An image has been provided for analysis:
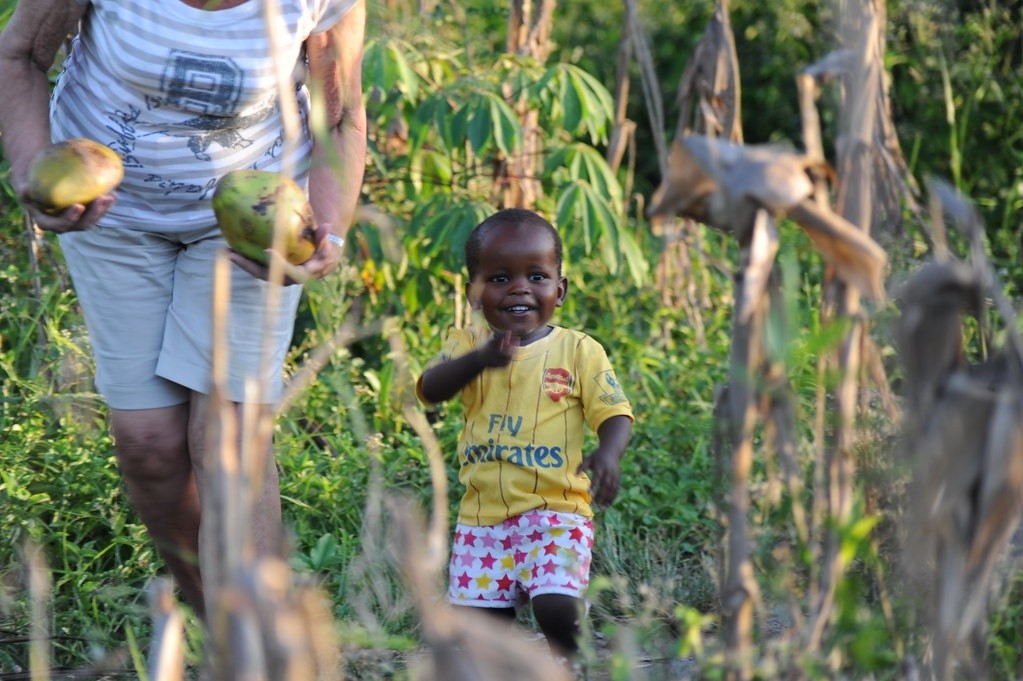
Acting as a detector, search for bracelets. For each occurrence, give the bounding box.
[329,233,346,248]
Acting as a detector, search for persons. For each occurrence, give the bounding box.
[414,207,635,681]
[1,0,369,681]
